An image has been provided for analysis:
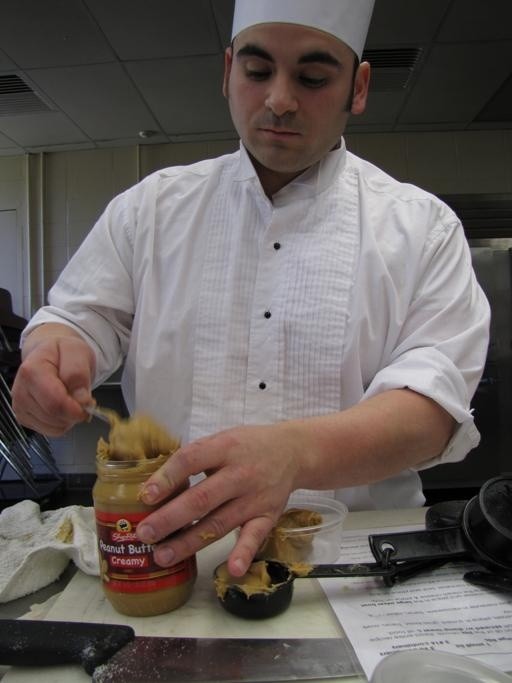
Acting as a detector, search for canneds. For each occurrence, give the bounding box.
[89,447,200,618]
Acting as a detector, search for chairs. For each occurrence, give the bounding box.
[0,288,67,503]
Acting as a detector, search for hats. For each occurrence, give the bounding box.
[231,0,377,65]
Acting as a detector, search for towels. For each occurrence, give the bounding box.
[0,498,101,605]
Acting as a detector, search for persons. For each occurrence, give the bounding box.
[11,22,493,576]
